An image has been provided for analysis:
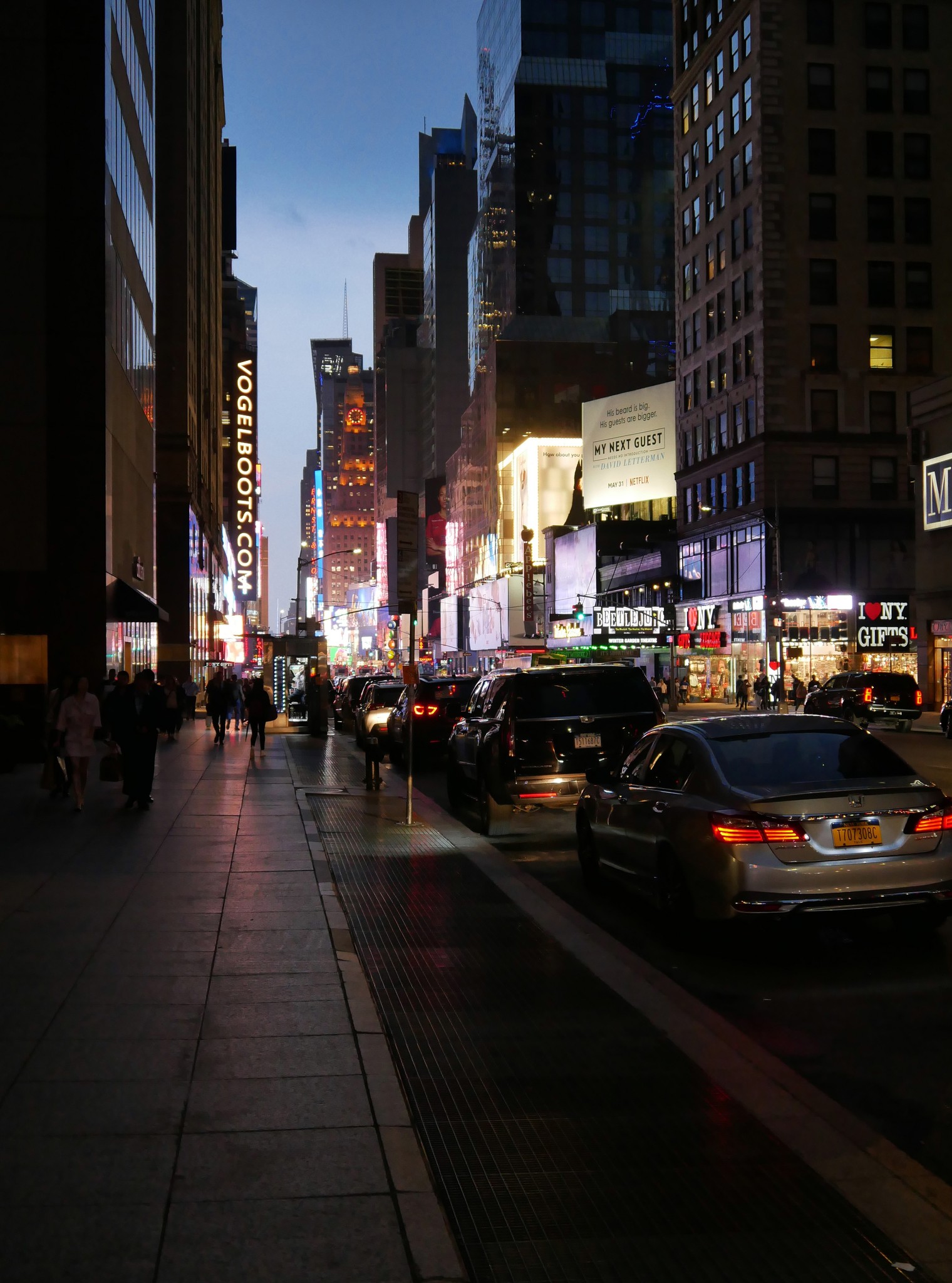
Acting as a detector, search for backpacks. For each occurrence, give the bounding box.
[228,686,238,701]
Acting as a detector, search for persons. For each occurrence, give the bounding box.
[96,669,186,811]
[736,675,743,707]
[663,676,690,706]
[649,677,657,694]
[182,675,199,721]
[56,676,102,811]
[754,675,772,710]
[772,678,781,709]
[657,679,667,703]
[808,675,821,693]
[245,678,271,758]
[288,666,309,719]
[739,680,752,710]
[204,671,236,746]
[203,672,257,730]
[365,724,385,789]
[791,674,800,706]
[795,681,808,713]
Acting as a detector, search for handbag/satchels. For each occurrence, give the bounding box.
[206,703,211,716]
[42,757,68,791]
[100,752,124,782]
[265,704,277,722]
[205,715,212,728]
[167,691,177,708]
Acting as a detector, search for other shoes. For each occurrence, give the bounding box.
[219,740,224,745]
[260,750,266,757]
[214,732,220,743]
[50,791,58,799]
[250,748,254,758]
[226,720,230,730]
[241,724,246,727]
[62,789,70,797]
[234,726,239,730]
[74,797,84,810]
[138,796,154,810]
[125,795,135,809]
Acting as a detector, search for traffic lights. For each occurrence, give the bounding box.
[786,646,803,658]
[572,604,583,621]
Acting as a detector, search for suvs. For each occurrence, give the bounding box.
[386,672,484,788]
[332,672,406,752]
[803,669,925,733]
[448,661,668,837]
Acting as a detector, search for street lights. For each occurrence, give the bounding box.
[295,542,362,636]
[466,596,504,668]
[504,574,547,650]
[697,503,790,714]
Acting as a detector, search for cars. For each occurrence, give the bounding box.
[939,700,952,739]
[576,713,952,964]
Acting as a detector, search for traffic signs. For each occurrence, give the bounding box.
[497,646,506,650]
[463,653,472,656]
[665,630,681,636]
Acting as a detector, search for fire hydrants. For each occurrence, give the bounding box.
[362,736,390,792]
